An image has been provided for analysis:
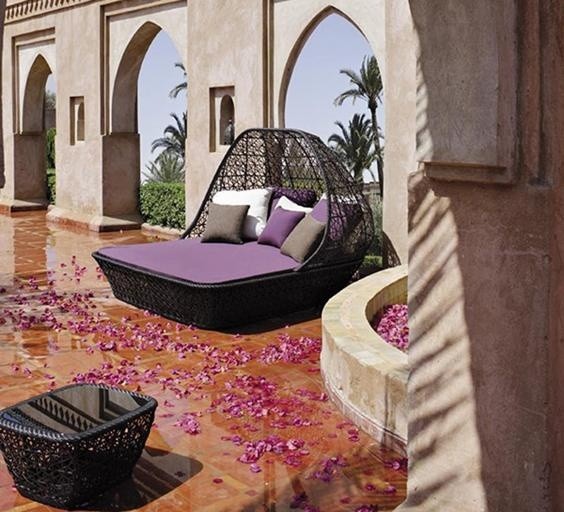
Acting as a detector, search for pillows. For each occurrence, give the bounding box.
[199,182,368,263]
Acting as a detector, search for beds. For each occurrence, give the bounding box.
[90,129,374,330]
